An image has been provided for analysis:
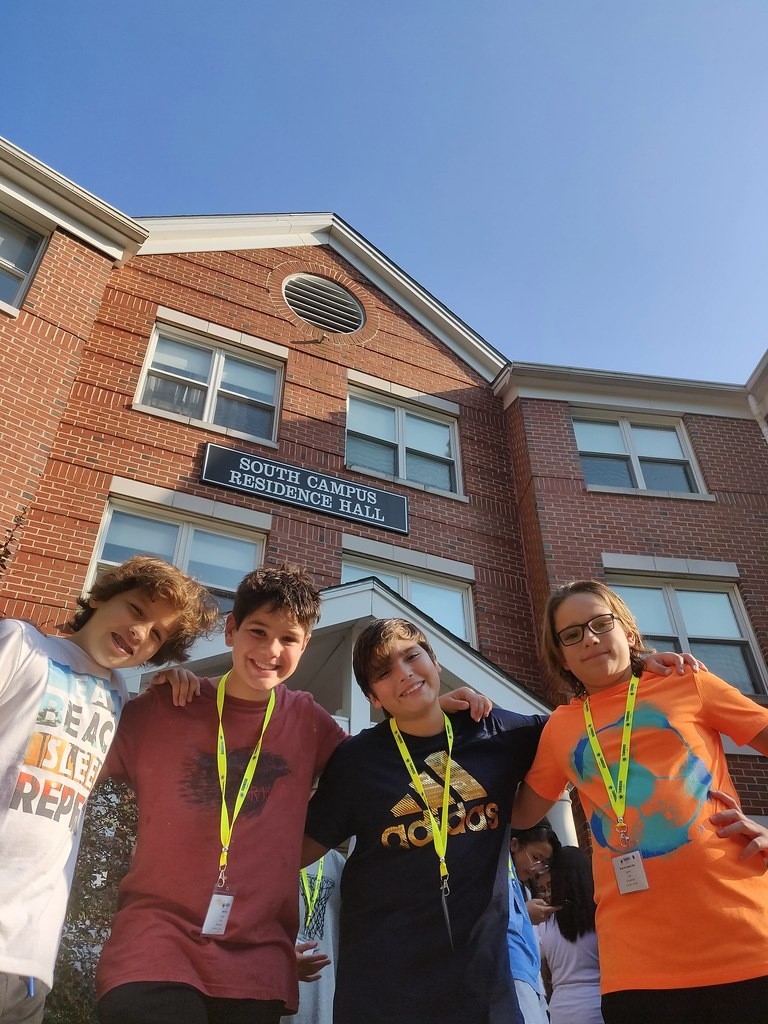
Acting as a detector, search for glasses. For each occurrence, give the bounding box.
[521,845,551,876]
[556,612,627,646]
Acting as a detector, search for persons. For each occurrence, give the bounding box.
[509,825,603,1024]
[280,770,346,1023]
[0,556,219,1024]
[95,565,492,1024]
[510,581,768,1024]
[300,618,709,1024]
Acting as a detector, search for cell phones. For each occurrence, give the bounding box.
[547,899,572,906]
